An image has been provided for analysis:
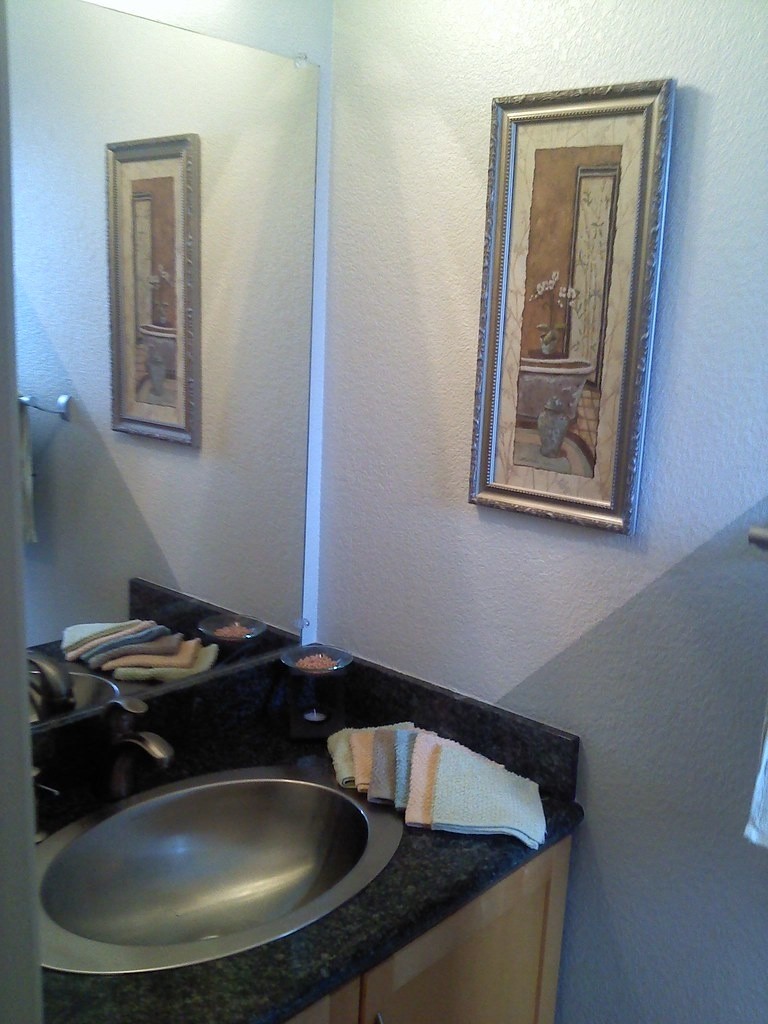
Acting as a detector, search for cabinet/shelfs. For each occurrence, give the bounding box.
[284,832,574,1024]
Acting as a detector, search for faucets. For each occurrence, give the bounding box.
[97,695,174,797]
[29,651,77,718]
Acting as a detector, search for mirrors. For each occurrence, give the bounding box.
[1,0,320,729]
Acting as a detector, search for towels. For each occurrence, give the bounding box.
[60,617,219,681]
[327,728,354,787]
[431,742,547,848]
[367,727,393,803]
[393,724,414,810]
[21,393,36,544]
[403,729,507,831]
[352,729,374,792]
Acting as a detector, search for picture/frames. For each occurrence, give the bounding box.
[105,133,203,448]
[466,77,677,536]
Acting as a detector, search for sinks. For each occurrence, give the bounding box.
[36,766,404,975]
[31,669,119,720]
